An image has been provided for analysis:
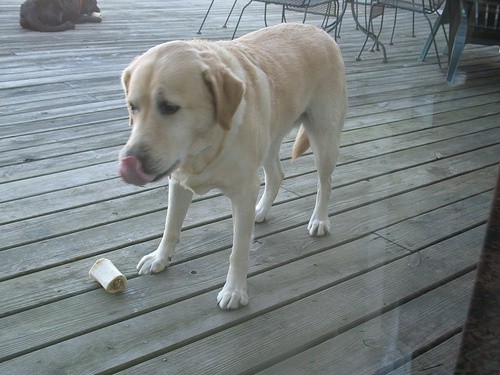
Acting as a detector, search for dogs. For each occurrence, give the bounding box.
[117,22,344,311]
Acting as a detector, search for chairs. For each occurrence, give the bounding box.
[195,0,500,86]
